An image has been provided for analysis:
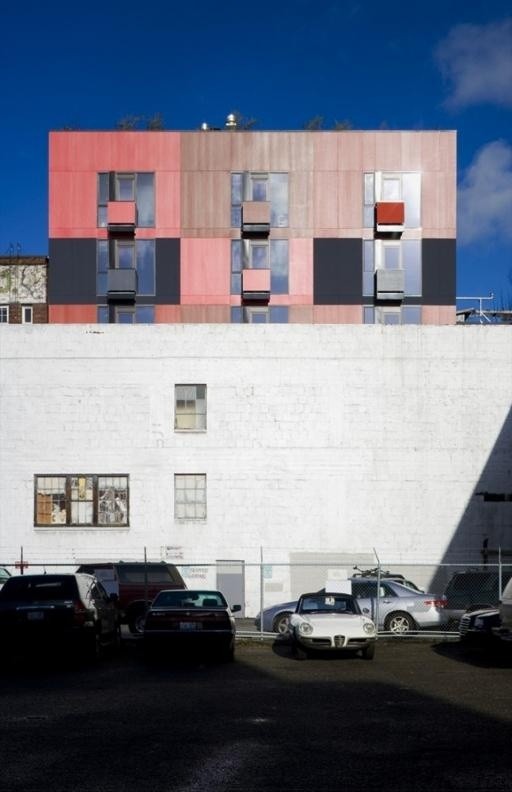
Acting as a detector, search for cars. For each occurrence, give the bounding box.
[256,565,512,661]
[0,561,235,665]
